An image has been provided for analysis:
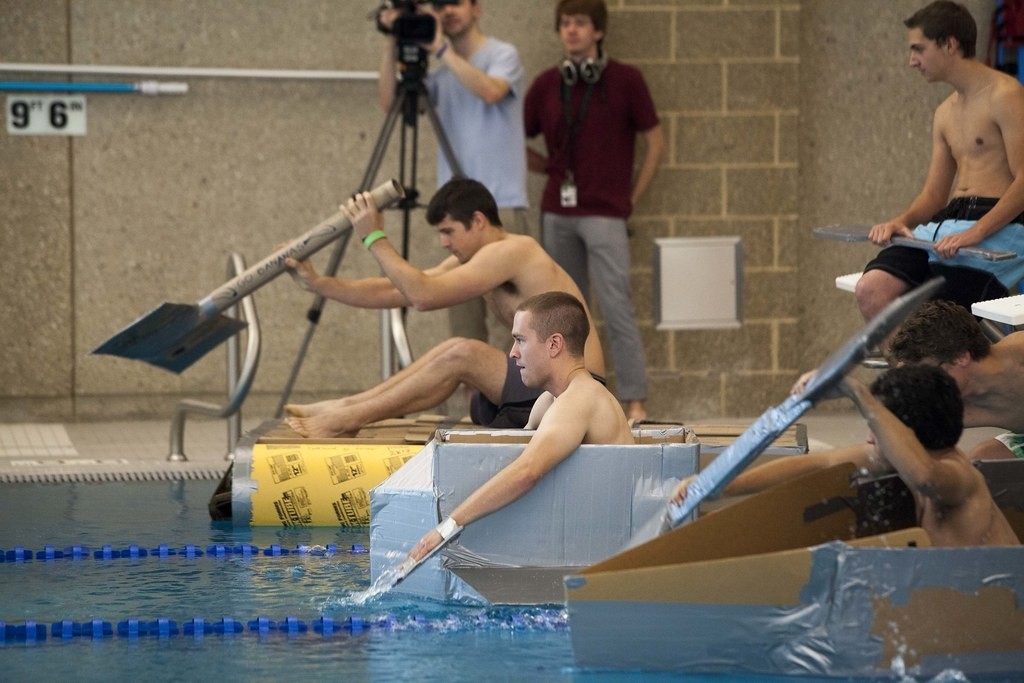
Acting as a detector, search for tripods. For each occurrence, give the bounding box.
[273,45,465,420]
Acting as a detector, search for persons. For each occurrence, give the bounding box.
[395,291,635,569]
[523,0,664,427]
[890,301,1024,459]
[275,178,607,439]
[855,0,1024,369]
[670,364,1021,547]
[378,0,530,403]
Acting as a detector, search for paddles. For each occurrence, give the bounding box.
[813,223,1017,263]
[619,275,946,554]
[88,175,408,379]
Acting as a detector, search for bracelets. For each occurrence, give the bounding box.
[437,44,447,59]
[365,231,386,248]
